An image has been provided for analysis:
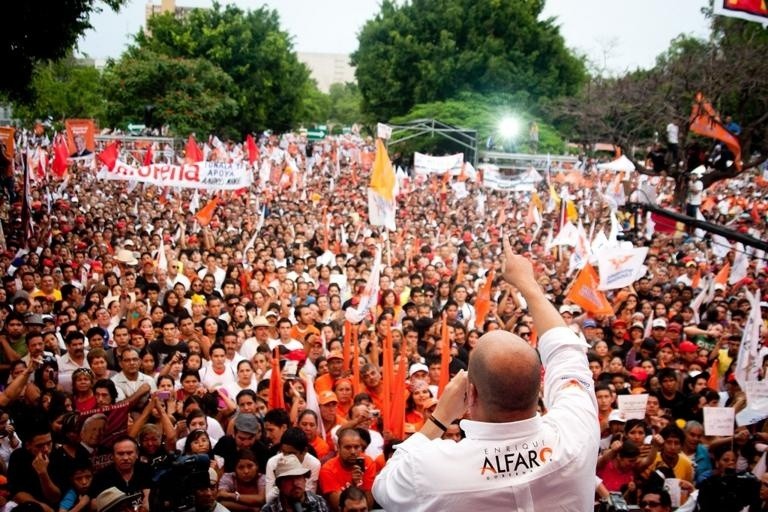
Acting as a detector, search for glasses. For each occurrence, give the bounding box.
[639,500,663,508]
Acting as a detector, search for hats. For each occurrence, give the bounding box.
[191,294,208,307]
[246,311,280,331]
[559,304,597,329]
[628,320,644,331]
[316,390,339,406]
[409,362,429,377]
[652,318,667,329]
[273,455,311,484]
[326,350,345,361]
[608,409,628,422]
[234,413,261,434]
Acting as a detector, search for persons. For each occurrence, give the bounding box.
[0,113,768,511]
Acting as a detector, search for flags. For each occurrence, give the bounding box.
[687,91,744,173]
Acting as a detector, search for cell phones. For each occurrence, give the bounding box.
[356,457,364,470]
[48,368,54,380]
[159,391,171,400]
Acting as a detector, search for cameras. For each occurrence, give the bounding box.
[180,353,188,361]
[371,410,380,418]
